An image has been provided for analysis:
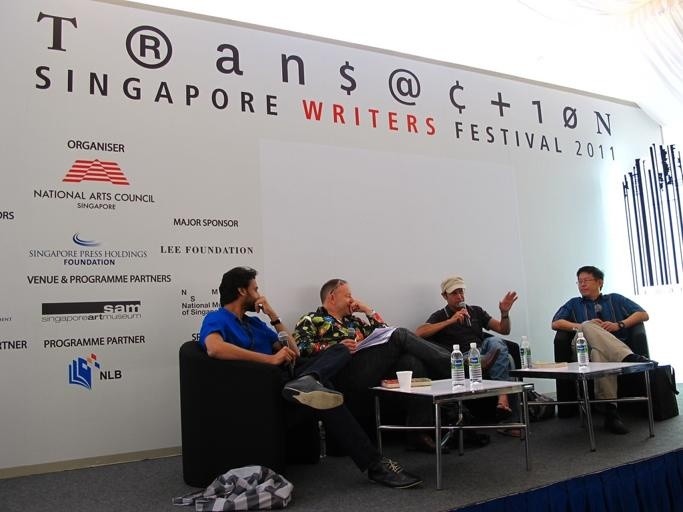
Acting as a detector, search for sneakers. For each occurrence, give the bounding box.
[496,421,529,436]
[497,402,513,415]
[636,355,658,370]
[603,413,630,434]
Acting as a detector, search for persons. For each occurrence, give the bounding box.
[291,278,501,451]
[551,265,659,434]
[416,275,532,437]
[199,265,425,490]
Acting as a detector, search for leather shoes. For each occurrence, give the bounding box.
[366,456,423,489]
[417,433,450,456]
[283,376,344,411]
[465,348,500,376]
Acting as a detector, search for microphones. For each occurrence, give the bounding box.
[459,302,472,329]
[348,328,356,339]
[594,304,605,322]
[278,331,294,380]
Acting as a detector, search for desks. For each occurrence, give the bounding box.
[369,377,534,490]
[509,361,656,452]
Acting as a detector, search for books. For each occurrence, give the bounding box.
[380,377,432,387]
[530,360,568,369]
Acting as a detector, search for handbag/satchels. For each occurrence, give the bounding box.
[522,385,555,421]
[172,464,293,511]
[635,365,679,421]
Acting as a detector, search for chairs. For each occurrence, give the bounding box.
[554,318,651,420]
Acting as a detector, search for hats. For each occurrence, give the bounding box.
[441,276,467,295]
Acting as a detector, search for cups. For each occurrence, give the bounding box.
[396,371,413,391]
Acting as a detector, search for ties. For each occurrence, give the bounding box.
[593,300,603,320]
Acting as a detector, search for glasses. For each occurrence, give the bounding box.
[576,279,594,285]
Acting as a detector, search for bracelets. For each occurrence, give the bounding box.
[501,316,508,318]
[270,317,281,325]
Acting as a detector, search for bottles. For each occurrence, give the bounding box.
[576,332,589,367]
[468,343,483,384]
[450,344,466,388]
[518,335,532,369]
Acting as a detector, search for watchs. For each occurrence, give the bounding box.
[617,321,625,329]
